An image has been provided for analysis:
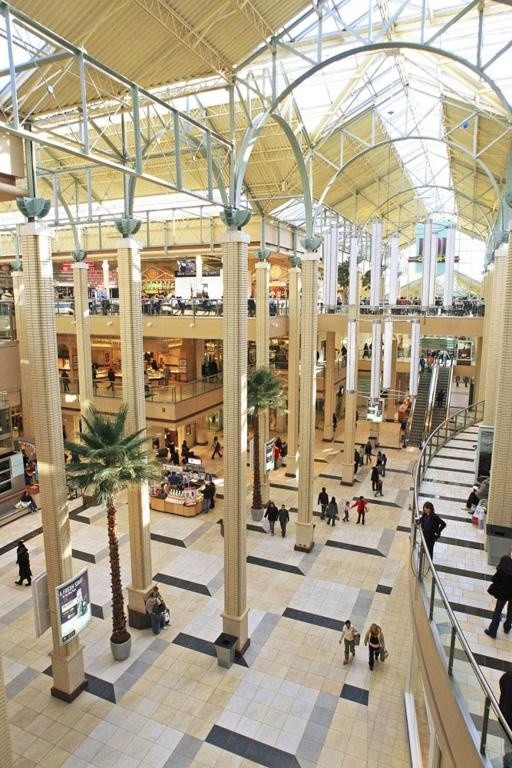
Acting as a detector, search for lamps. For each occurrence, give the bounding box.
[320,79,458,407]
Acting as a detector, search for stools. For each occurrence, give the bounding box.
[26,484,39,494]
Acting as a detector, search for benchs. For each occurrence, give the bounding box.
[0,488,33,527]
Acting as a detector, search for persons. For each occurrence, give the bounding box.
[154,586,171,630]
[339,620,359,665]
[364,624,385,671]
[415,501,446,567]
[14,540,33,586]
[484,552,512,639]
[145,592,161,635]
[498,670,512,768]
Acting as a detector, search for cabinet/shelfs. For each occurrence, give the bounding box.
[0,388,23,451]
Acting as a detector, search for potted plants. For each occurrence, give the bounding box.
[361,269,378,304]
[246,365,289,521]
[337,252,363,314]
[63,399,167,661]
